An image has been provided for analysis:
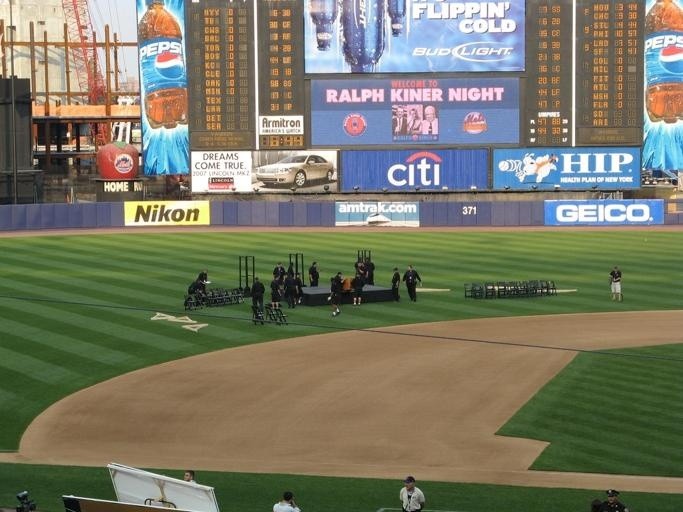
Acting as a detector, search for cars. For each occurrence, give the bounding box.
[256,154,335,187]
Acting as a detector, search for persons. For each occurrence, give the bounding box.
[419,105,438,136]
[350,257,375,305]
[403,264,421,302]
[188,268,208,306]
[399,476,425,512]
[608,266,622,302]
[392,107,407,134]
[327,277,339,317]
[591,500,603,512]
[184,470,196,483]
[391,268,401,302]
[250,277,265,319]
[407,109,420,134]
[271,262,307,314]
[272,492,301,512]
[309,261,319,287]
[603,489,627,511]
[336,272,346,291]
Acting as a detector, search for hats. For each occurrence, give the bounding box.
[606,489,618,497]
[284,492,293,499]
[403,479,413,483]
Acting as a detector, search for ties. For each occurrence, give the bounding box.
[428,122,432,133]
[411,119,415,128]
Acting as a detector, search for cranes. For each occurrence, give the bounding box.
[61,0,127,146]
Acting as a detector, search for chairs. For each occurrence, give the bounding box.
[265,305,289,326]
[251,306,264,325]
[464,279,558,299]
[184,288,246,310]
